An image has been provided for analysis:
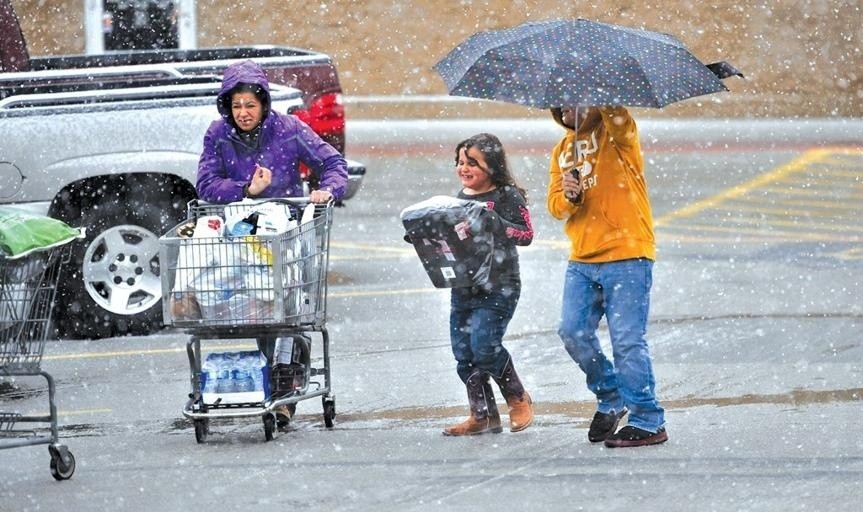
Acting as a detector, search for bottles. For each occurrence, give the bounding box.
[202,350,267,395]
[170,291,201,319]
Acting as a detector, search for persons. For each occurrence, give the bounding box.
[548,104,668,445]
[441,134,534,435]
[195,62,347,422]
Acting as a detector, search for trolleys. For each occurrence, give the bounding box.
[0,240,78,480]
[157,196,337,442]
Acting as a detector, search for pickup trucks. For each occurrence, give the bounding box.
[0,0,346,162]
[0,83,366,335]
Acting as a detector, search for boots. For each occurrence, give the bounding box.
[442,377,504,437]
[486,354,535,432]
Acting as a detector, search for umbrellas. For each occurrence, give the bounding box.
[431,14,744,201]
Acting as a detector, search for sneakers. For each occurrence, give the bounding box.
[272,403,298,429]
[603,425,670,448]
[588,408,629,444]
